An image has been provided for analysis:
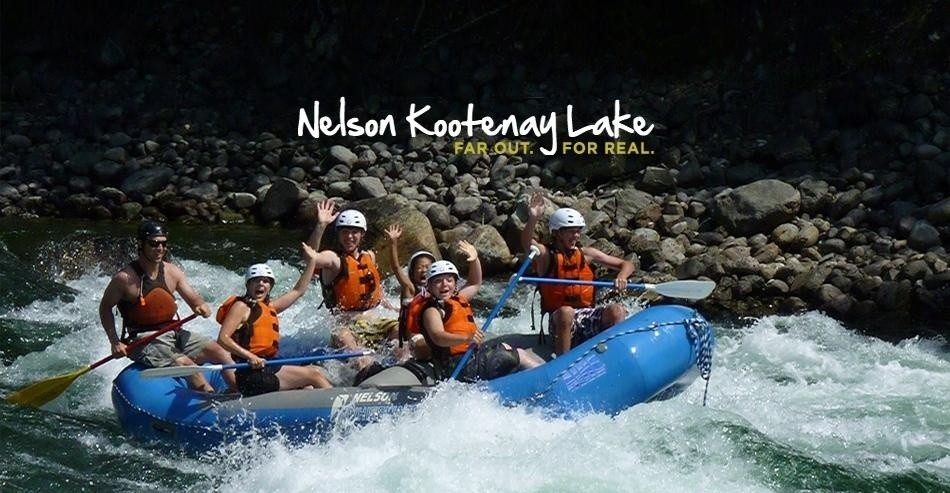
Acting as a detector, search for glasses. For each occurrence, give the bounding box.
[145,237,170,248]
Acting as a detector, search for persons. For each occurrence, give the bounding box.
[215,240,331,396]
[422,238,543,380]
[302,198,412,370]
[520,191,637,358]
[100,220,238,401]
[382,223,438,341]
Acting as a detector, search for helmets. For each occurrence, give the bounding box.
[334,209,367,232]
[548,207,586,235]
[245,262,276,287]
[136,220,169,238]
[408,250,461,284]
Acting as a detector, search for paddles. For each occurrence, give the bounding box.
[6,307,205,408]
[510,273,715,301]
[143,348,376,379]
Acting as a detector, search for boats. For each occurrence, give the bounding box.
[110,303,711,463]
